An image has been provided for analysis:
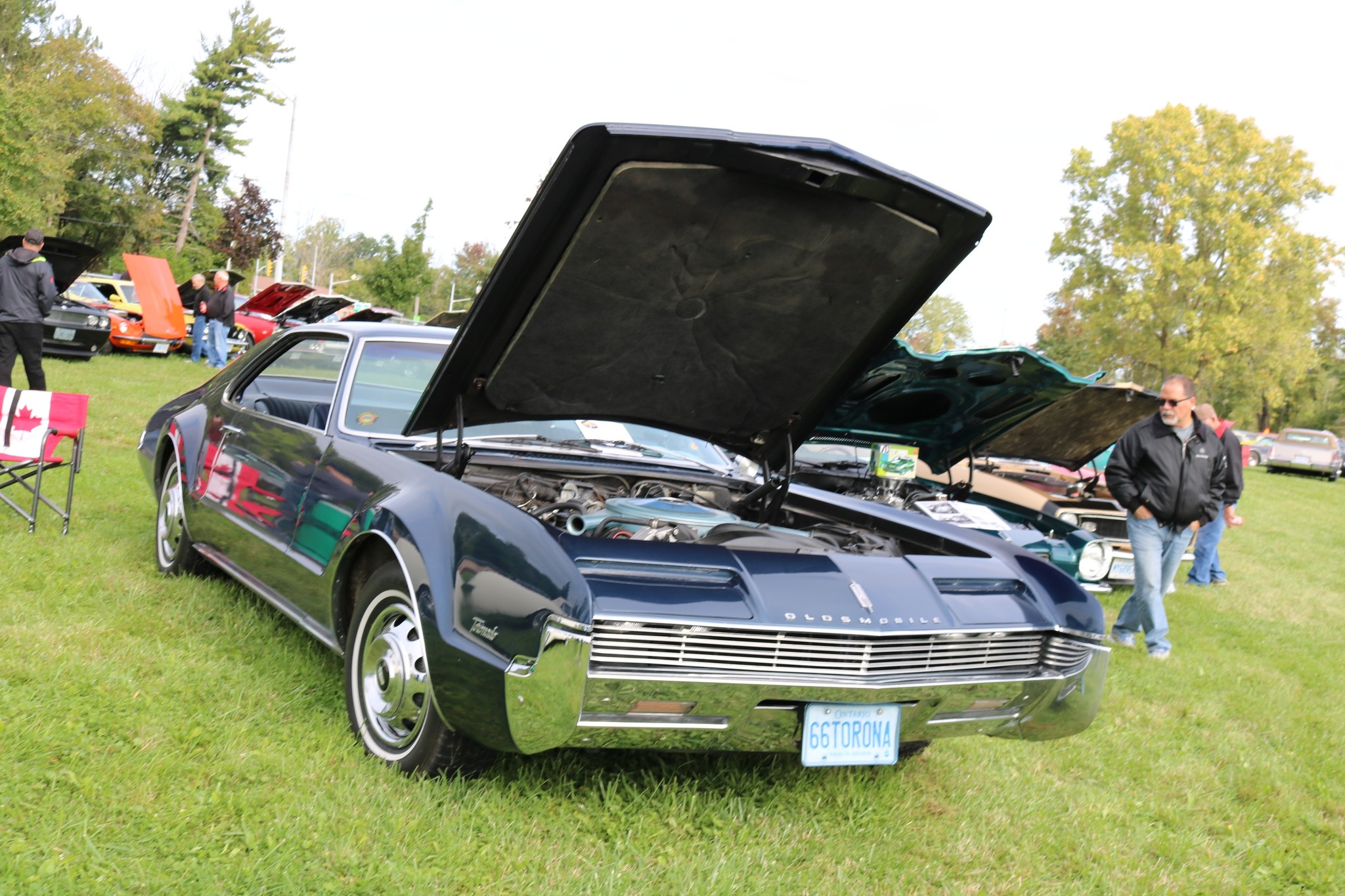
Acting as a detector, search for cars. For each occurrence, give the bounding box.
[136,122,1113,783]
[1232,428,1345,482]
[0,236,468,370]
[787,337,1195,595]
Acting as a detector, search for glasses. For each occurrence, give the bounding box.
[1155,396,1193,407]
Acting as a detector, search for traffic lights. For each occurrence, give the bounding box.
[266,259,274,276]
[301,265,308,283]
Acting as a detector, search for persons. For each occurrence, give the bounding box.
[1181,403,1244,586]
[186,275,213,365]
[0,230,56,392]
[1102,374,1227,660]
[199,270,235,370]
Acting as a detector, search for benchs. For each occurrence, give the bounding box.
[257,394,411,435]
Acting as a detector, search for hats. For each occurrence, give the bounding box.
[25,228,44,245]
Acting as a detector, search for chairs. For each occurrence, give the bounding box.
[0,385,92,536]
